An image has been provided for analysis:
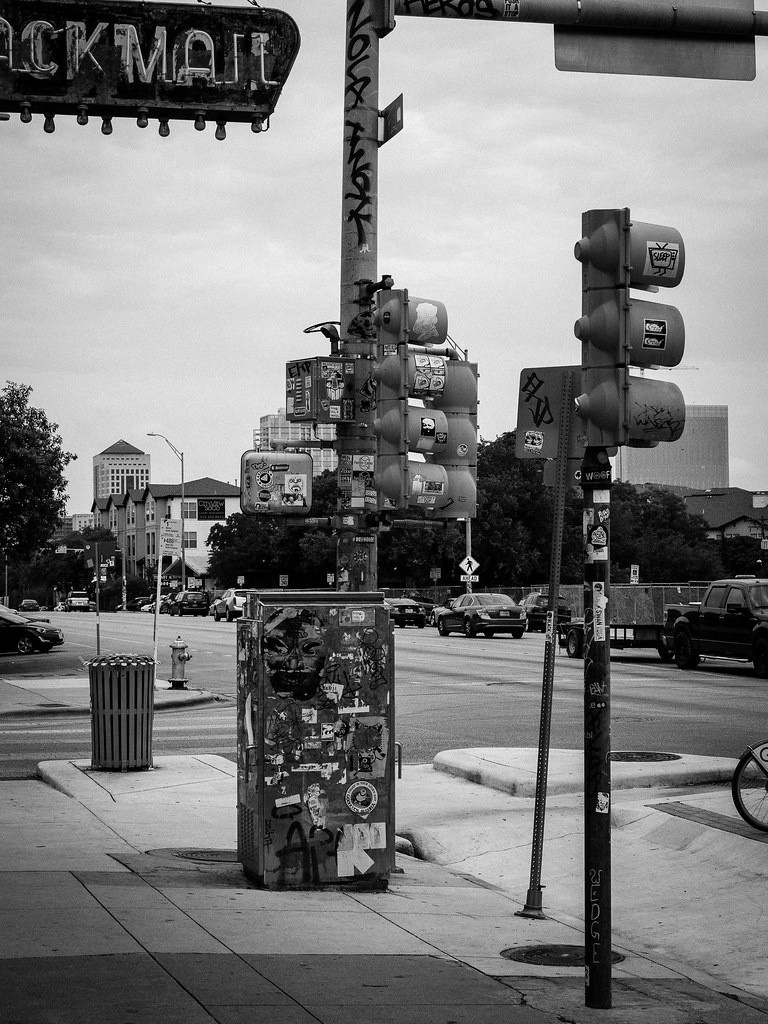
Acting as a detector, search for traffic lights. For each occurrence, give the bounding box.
[571,207,688,449]
[368,288,449,513]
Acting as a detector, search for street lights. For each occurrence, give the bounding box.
[146,431,187,592]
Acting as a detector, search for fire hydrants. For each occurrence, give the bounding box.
[167,635,193,690]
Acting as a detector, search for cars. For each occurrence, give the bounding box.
[435,591,527,640]
[39,598,96,612]
[208,598,217,616]
[18,599,40,612]
[429,598,458,627]
[385,597,426,628]
[0,602,65,655]
[114,592,178,614]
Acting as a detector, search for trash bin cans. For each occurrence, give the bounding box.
[86,652,155,772]
[235,589,395,893]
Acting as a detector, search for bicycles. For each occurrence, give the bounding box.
[730,738,768,833]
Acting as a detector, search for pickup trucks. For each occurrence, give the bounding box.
[661,578,768,680]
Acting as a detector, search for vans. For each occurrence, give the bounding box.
[64,591,90,612]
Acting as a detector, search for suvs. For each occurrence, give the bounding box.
[168,590,209,617]
[213,587,259,623]
[519,591,572,632]
